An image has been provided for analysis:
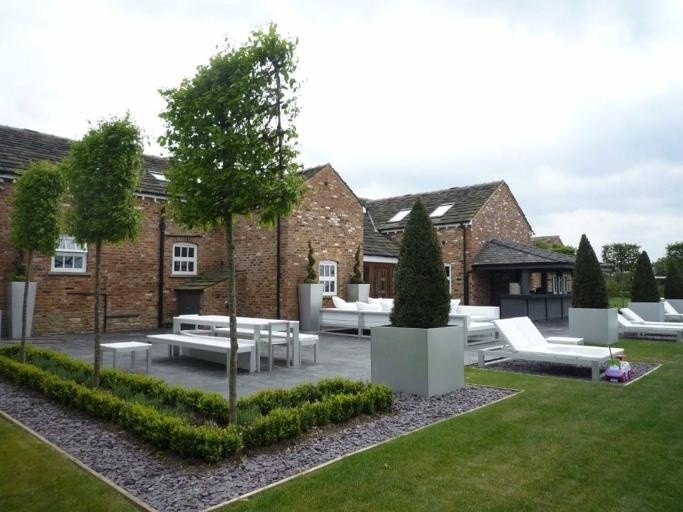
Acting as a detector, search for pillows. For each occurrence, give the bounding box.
[356,301,381,311]
[446,298,461,313]
[381,299,394,310]
[332,294,349,309]
[368,296,382,304]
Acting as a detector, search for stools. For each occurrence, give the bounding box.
[100,339,152,376]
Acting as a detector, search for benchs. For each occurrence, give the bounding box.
[213,315,319,367]
[320,305,501,346]
[146,320,259,372]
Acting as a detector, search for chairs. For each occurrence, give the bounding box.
[261,321,290,371]
[660,297,683,319]
[478,316,623,384]
[617,313,683,342]
[619,308,683,327]
[172,314,212,335]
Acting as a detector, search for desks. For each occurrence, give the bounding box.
[172,314,300,371]
